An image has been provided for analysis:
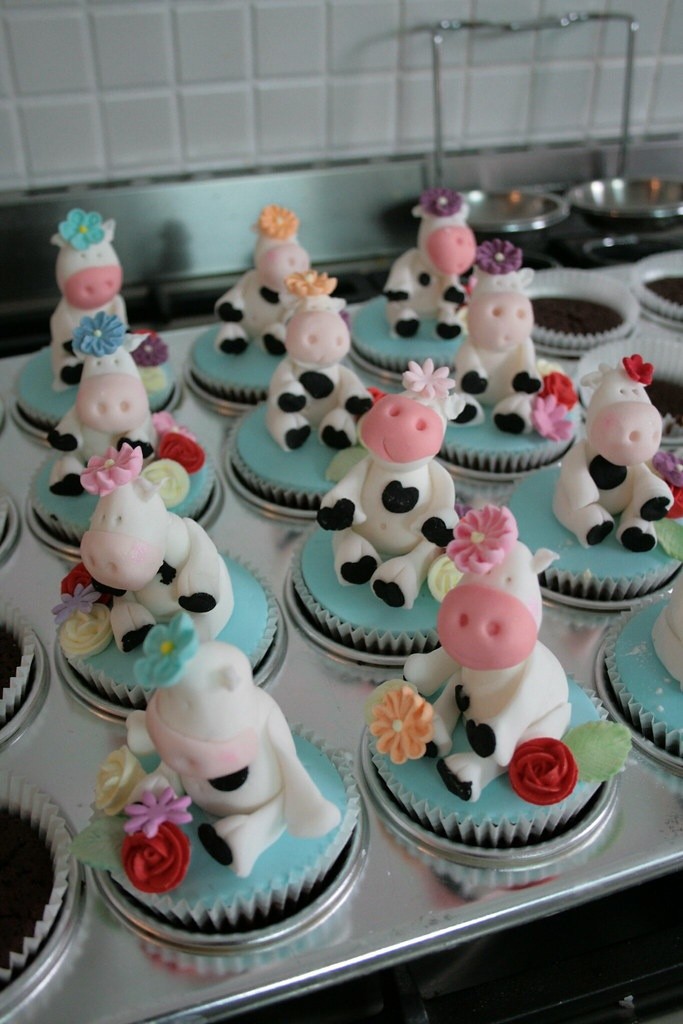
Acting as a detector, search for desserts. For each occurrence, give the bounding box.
[0,193,683,986]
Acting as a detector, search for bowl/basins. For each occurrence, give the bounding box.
[567,178,683,235]
[447,189,569,254]
